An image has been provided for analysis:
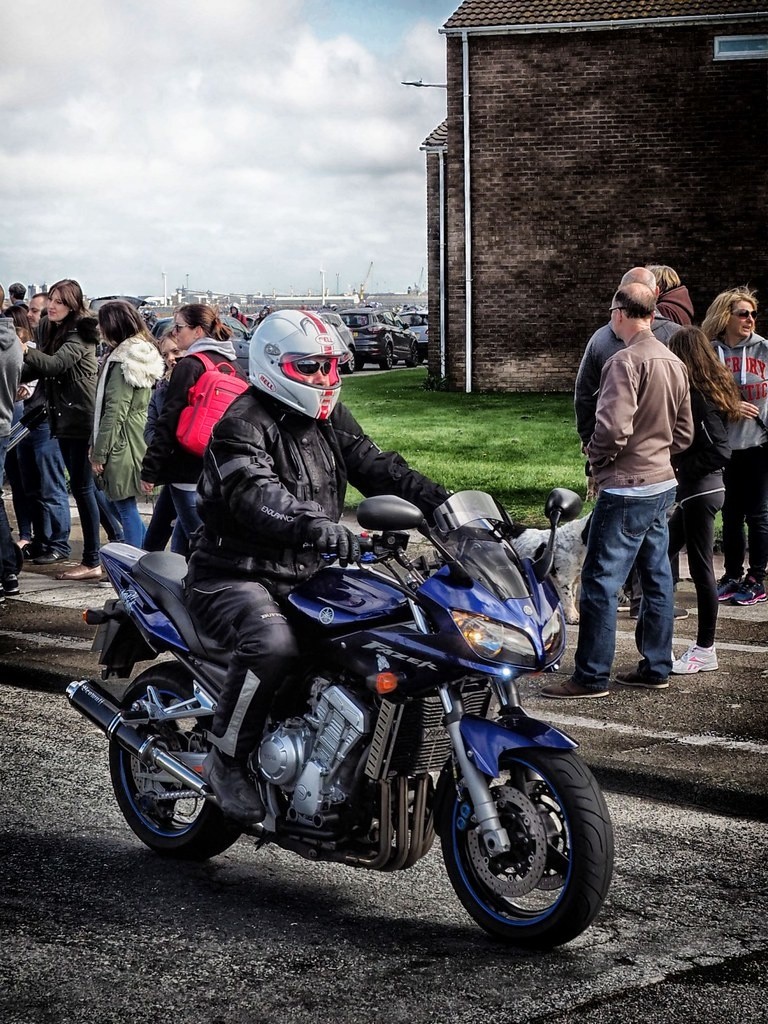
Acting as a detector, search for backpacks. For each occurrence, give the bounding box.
[177,353,249,456]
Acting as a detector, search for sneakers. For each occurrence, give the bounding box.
[730,573,768,606]
[32,548,70,564]
[671,644,719,673]
[716,571,743,601]
[617,592,632,612]
[18,543,43,562]
[202,745,265,823]
[0,573,20,602]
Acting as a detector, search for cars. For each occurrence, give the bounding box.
[339,308,420,369]
[399,310,428,365]
[89,295,252,342]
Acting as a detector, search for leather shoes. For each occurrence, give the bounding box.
[55,564,102,580]
[539,676,610,698]
[614,667,671,688]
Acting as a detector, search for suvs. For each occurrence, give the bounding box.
[306,308,355,375]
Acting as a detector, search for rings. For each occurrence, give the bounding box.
[92,467,97,469]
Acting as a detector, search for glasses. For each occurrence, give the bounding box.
[175,324,194,332]
[609,306,626,314]
[294,359,332,376]
[731,309,757,319]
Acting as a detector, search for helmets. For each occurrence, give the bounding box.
[230,303,241,314]
[248,308,343,420]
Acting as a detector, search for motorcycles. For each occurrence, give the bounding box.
[64,489,619,950]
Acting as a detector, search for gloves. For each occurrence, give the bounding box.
[307,517,362,568]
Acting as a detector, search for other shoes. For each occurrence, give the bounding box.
[629,603,690,619]
[99,576,114,588]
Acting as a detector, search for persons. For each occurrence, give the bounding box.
[653,327,741,676]
[0,276,272,597]
[572,263,768,621]
[539,281,695,700]
[179,308,499,825]
